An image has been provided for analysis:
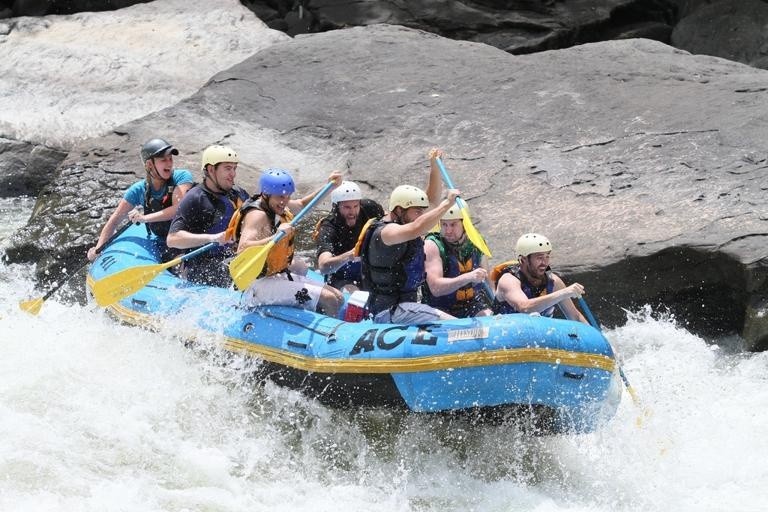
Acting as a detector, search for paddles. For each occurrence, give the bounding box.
[435,155,492,258]
[228,177,337,292]
[19,217,140,316]
[93,242,219,307]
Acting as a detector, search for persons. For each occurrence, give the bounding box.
[424,198,494,317]
[166,145,250,289]
[352,147,462,325]
[223,168,346,319]
[488,232,589,327]
[87,137,198,277]
[313,180,386,294]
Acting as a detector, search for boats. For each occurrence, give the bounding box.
[75,208,626,438]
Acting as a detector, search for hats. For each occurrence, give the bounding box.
[154,147,180,156]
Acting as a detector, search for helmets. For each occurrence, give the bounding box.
[141,138,172,162]
[390,185,429,211]
[515,232,553,262]
[440,197,470,220]
[201,144,240,171]
[333,181,363,202]
[260,170,295,194]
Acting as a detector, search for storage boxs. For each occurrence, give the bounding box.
[347,290,371,323]
